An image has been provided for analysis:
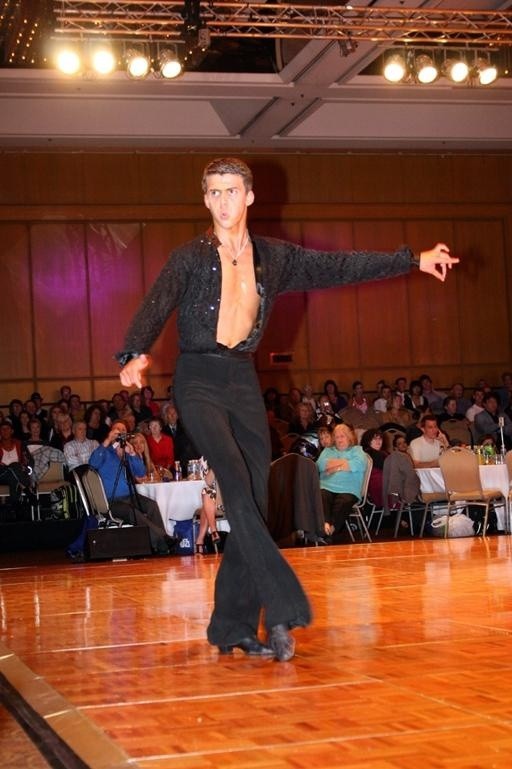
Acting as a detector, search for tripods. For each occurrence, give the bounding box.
[103,453,155,551]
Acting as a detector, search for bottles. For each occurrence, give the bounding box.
[173,457,205,481]
[460,443,506,465]
[148,464,155,482]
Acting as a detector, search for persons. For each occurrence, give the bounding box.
[263,374,511,544]
[1,386,224,560]
[109,155,463,663]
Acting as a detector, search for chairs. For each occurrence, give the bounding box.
[364,467,412,538]
[335,453,372,543]
[438,445,507,538]
[384,452,457,536]
[0,446,70,519]
[68,464,136,559]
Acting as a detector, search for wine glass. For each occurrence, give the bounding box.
[158,465,165,483]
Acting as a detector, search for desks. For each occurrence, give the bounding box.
[132,479,222,555]
[409,459,511,534]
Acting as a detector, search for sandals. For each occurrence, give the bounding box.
[196,544,205,555]
[211,531,221,543]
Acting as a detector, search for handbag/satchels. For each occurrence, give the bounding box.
[441,513,475,537]
[51,484,84,521]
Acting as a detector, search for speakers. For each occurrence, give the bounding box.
[83,526,153,563]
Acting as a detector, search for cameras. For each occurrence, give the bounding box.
[115,431,135,448]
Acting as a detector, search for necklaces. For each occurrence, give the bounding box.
[213,231,250,266]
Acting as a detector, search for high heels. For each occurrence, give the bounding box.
[218,637,274,655]
[269,623,295,662]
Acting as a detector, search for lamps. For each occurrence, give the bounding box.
[50,41,184,79]
[376,43,500,86]
